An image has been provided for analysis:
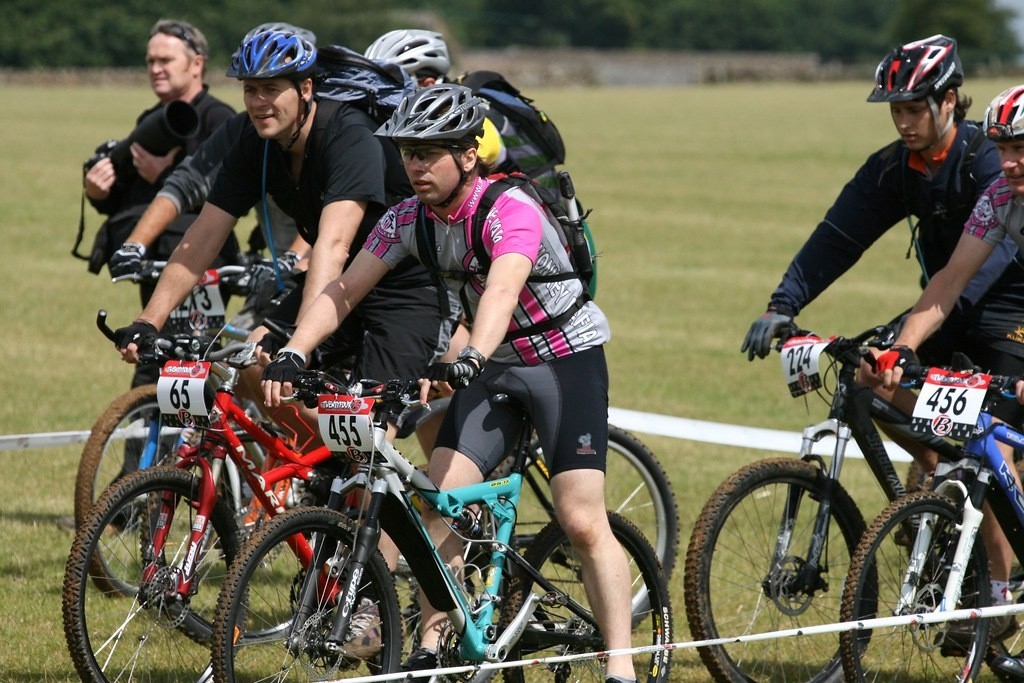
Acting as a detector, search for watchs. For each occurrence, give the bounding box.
[457,346,485,372]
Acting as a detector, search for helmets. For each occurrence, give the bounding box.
[373,83,486,148]
[364,29,451,75]
[225,23,317,81]
[866,33,963,102]
[982,85,1024,141]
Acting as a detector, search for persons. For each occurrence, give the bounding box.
[365,29,598,459]
[258,80,638,683]
[873,85,1024,683]
[57,18,238,536]
[115,23,462,662]
[740,35,1024,543]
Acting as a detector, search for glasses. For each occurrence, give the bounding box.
[160,23,199,55]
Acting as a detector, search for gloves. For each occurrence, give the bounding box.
[740,306,794,362]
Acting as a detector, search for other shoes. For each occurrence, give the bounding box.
[397,632,460,683]
[945,597,1018,644]
[603,675,640,683]
[57,517,123,539]
[892,445,965,546]
[274,472,315,510]
[332,598,381,658]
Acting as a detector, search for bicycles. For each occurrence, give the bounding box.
[208,365,676,683]
[838,343,1024,683]
[60,253,684,683]
[683,306,1024,683]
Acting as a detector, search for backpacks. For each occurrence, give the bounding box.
[416,172,597,299]
[454,70,565,202]
[313,45,418,125]
